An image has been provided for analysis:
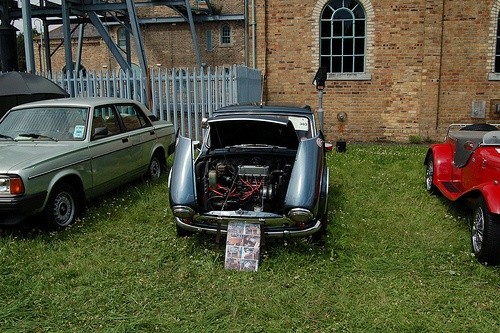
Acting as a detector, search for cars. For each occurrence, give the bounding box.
[168,104,332,249]
[0,97,176,233]
[424,123,499,267]
